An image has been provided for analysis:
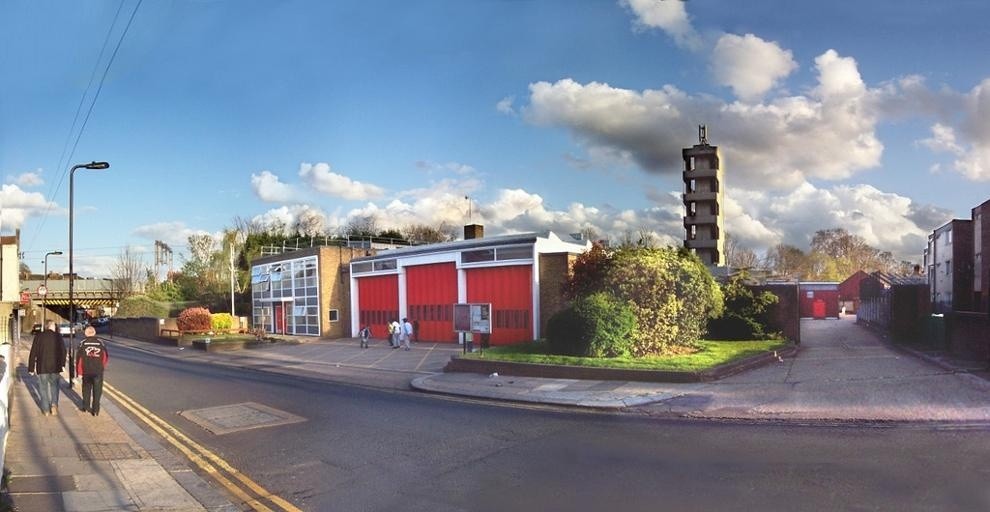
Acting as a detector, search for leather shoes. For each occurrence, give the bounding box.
[45,404,58,416]
[83,409,98,416]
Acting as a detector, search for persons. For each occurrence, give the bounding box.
[413,319,419,341]
[400,318,410,351]
[358,326,372,349]
[27,319,68,416]
[481,306,489,320]
[75,325,109,417]
[391,319,402,348]
[386,320,394,346]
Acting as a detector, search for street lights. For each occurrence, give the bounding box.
[69,160,110,379]
[43,252,62,332]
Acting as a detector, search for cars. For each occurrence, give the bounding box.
[56,323,75,337]
[75,316,110,331]
[32,324,41,335]
